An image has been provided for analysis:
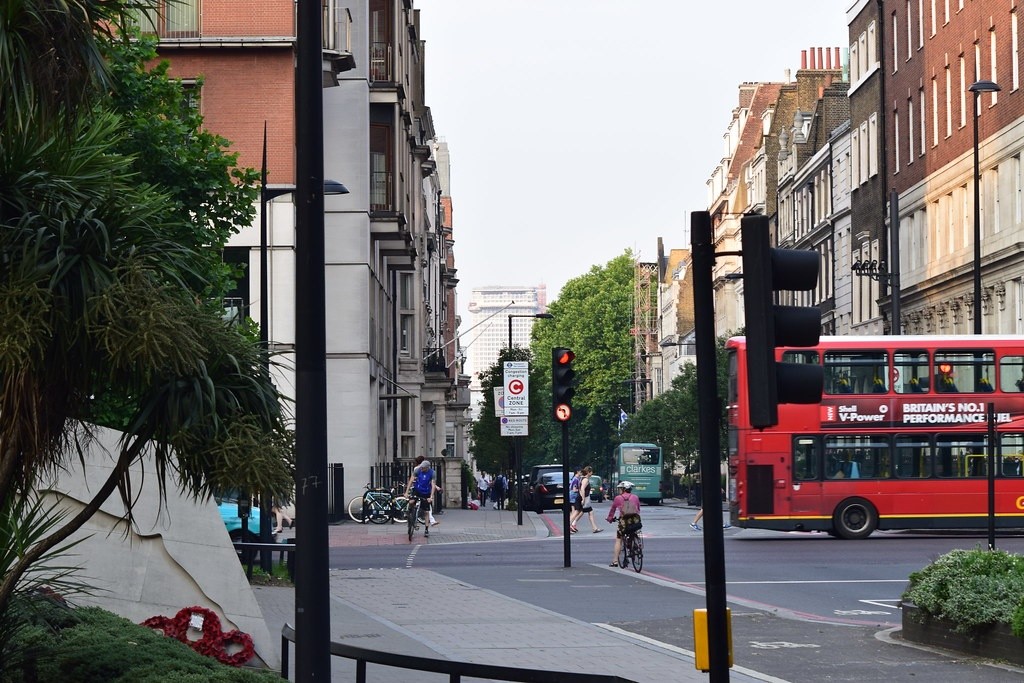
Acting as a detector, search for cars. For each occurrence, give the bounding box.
[215,495,277,561]
[578,475,605,503]
[530,471,574,514]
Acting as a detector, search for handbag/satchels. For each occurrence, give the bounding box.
[569,490,579,503]
[583,496,591,509]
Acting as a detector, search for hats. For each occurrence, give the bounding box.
[420,461,430,467]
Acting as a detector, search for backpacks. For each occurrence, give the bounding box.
[495,477,504,492]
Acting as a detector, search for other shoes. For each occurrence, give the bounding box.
[424,530,429,536]
[690,524,701,530]
[723,525,731,529]
[290,521,294,529]
[274,530,283,533]
[431,522,438,525]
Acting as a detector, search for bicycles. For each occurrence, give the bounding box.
[606,515,644,573]
[347,479,430,525]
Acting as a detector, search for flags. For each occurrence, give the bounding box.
[618,409,633,437]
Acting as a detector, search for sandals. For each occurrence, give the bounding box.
[609,561,618,567]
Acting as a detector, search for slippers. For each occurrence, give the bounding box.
[593,528,604,533]
[570,525,578,533]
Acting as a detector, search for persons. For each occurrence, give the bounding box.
[477,470,517,509]
[403,460,436,536]
[413,455,443,527]
[271,504,293,532]
[689,486,732,532]
[667,489,672,499]
[659,481,664,503]
[606,481,642,568]
[597,477,612,502]
[568,465,604,534]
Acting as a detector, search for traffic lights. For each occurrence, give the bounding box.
[740,214,827,432]
[552,347,575,406]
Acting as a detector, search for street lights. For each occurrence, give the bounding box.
[261,120,351,575]
[509,313,555,525]
[968,80,1003,473]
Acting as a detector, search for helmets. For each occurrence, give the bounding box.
[617,481,635,490]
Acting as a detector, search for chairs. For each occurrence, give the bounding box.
[824,366,1023,478]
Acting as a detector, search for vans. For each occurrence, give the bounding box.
[514,464,564,509]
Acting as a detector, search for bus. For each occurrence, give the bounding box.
[717,334,1024,541]
[608,442,665,507]
